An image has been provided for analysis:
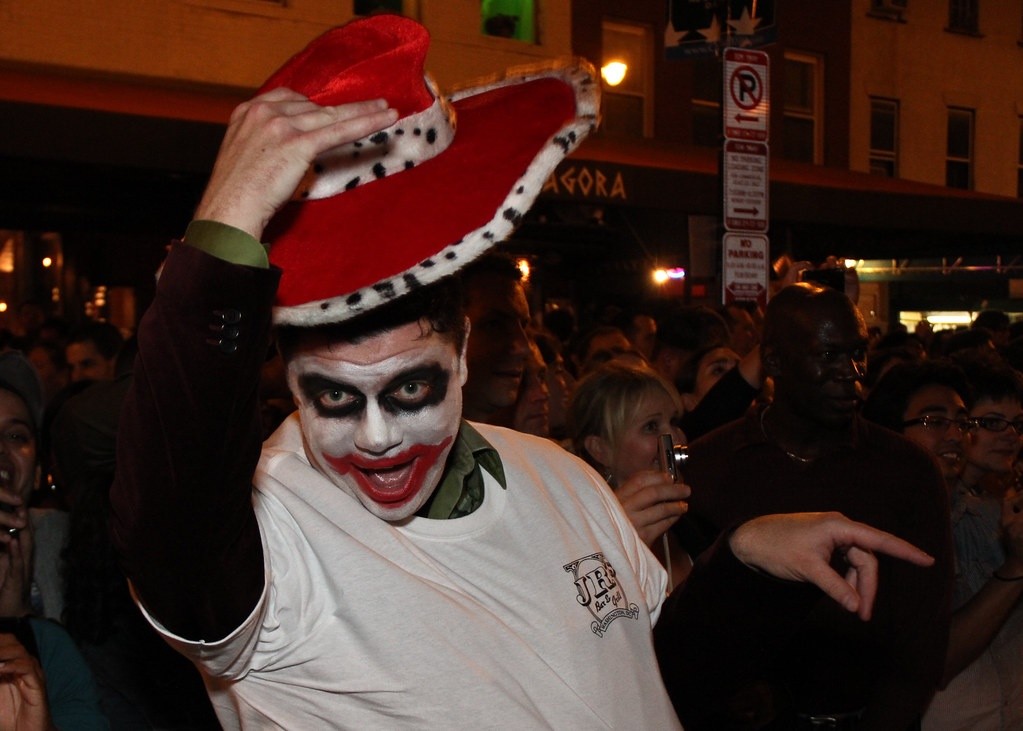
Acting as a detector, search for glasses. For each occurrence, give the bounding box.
[973,416,1023,435]
[899,415,979,435]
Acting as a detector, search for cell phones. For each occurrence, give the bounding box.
[1001,491,1023,529]
[0,501,19,555]
[801,269,846,295]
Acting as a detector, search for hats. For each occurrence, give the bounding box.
[154,15,603,325]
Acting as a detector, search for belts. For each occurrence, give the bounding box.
[792,707,865,731]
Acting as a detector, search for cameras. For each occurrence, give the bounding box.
[659,432,689,485]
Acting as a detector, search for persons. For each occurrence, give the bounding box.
[99,6,935,731]
[0,248,1019,730]
